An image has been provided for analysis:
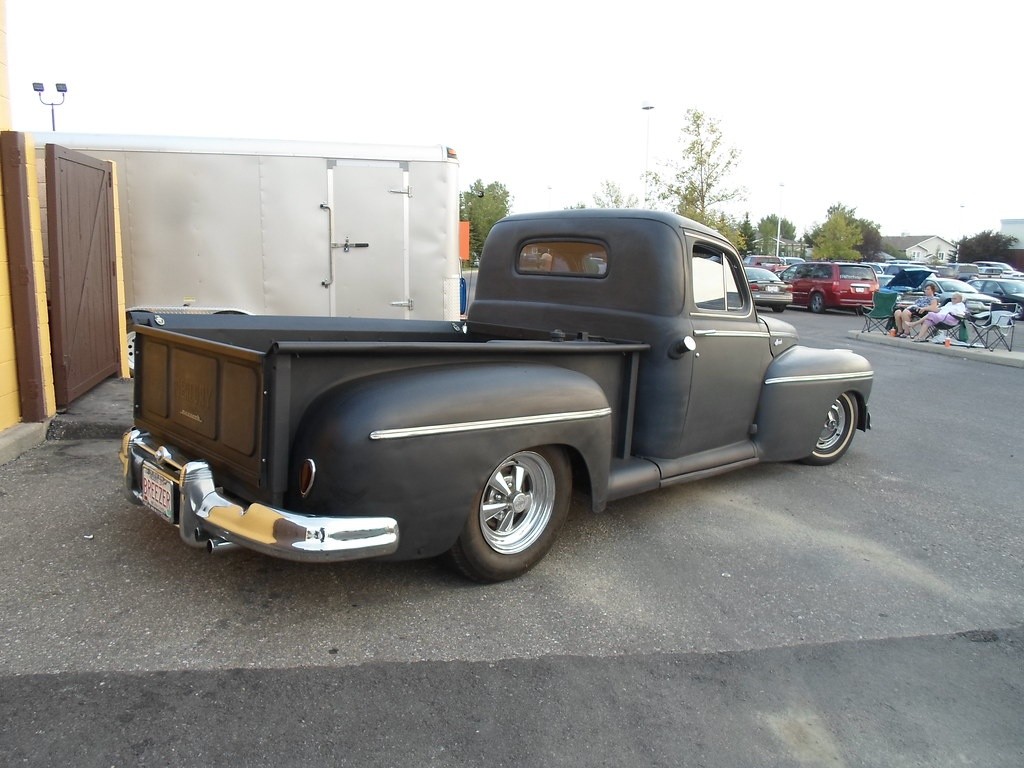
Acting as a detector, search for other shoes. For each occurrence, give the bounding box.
[896,329,910,338]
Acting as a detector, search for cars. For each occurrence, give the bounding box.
[740,253,1024,293]
[727,267,794,313]
[966,279,1023,321]
[921,278,1002,322]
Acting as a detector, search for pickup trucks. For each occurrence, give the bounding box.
[118,208,874,586]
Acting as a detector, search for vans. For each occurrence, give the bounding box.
[779,261,879,315]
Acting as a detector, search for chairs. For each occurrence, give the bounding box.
[925,298,969,340]
[968,302,1018,351]
[861,291,898,336]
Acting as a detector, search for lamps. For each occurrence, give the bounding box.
[33,82,67,131]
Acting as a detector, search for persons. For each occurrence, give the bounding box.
[904,292,965,340]
[536,247,553,271]
[894,283,938,338]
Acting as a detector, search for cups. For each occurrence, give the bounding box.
[945,339,951,348]
[890,329,895,336]
